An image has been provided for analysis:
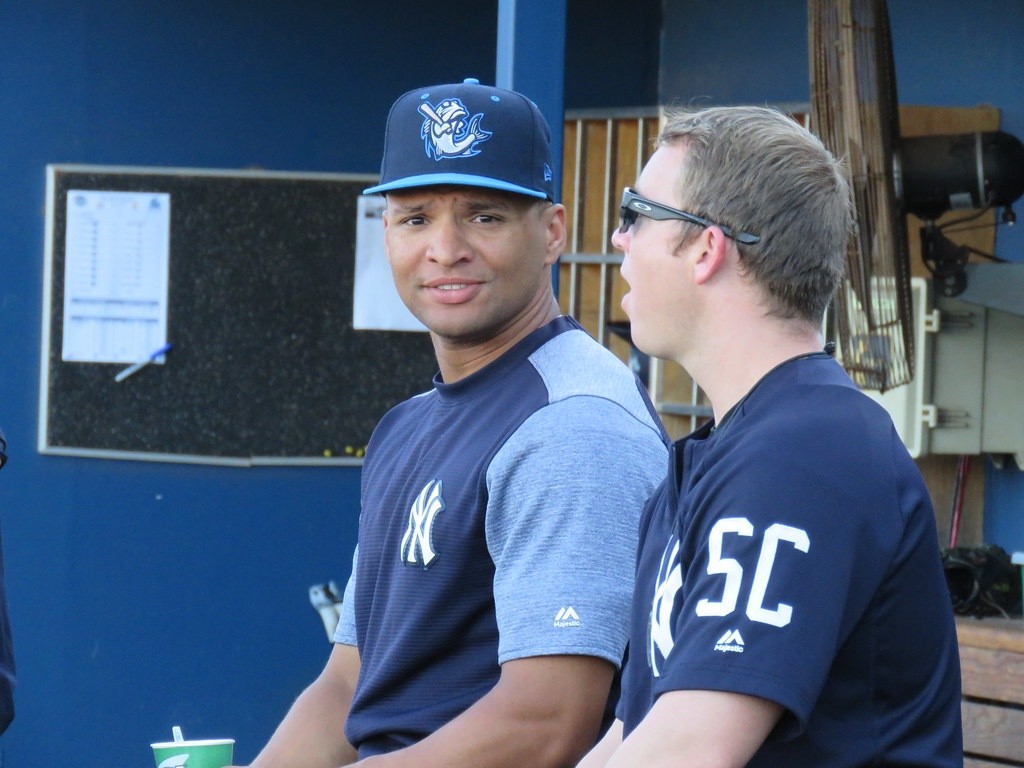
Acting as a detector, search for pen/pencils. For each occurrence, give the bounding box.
[115,342,172,384]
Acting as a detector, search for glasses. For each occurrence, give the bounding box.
[618,187,761,244]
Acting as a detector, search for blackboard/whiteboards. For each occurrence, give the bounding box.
[34,164,440,466]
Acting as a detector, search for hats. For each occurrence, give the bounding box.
[363,78,555,203]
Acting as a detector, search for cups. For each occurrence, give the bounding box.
[150,739,235,768]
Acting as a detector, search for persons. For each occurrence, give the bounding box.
[575,107,963,768]
[247,79,671,768]
